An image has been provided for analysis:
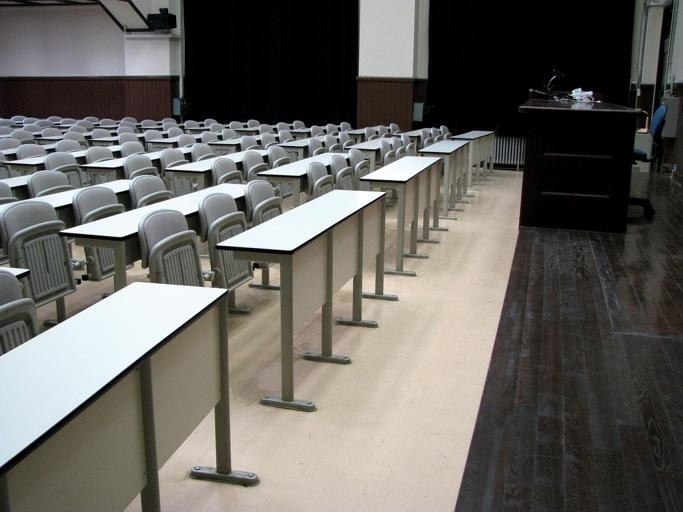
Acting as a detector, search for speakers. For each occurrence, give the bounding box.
[147,8,176,29]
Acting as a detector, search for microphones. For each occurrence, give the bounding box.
[551,67,565,79]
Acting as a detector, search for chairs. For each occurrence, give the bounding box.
[628,103,667,222]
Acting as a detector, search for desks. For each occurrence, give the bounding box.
[0,282,258,512]
[216,190,397,411]
[359,130,496,276]
